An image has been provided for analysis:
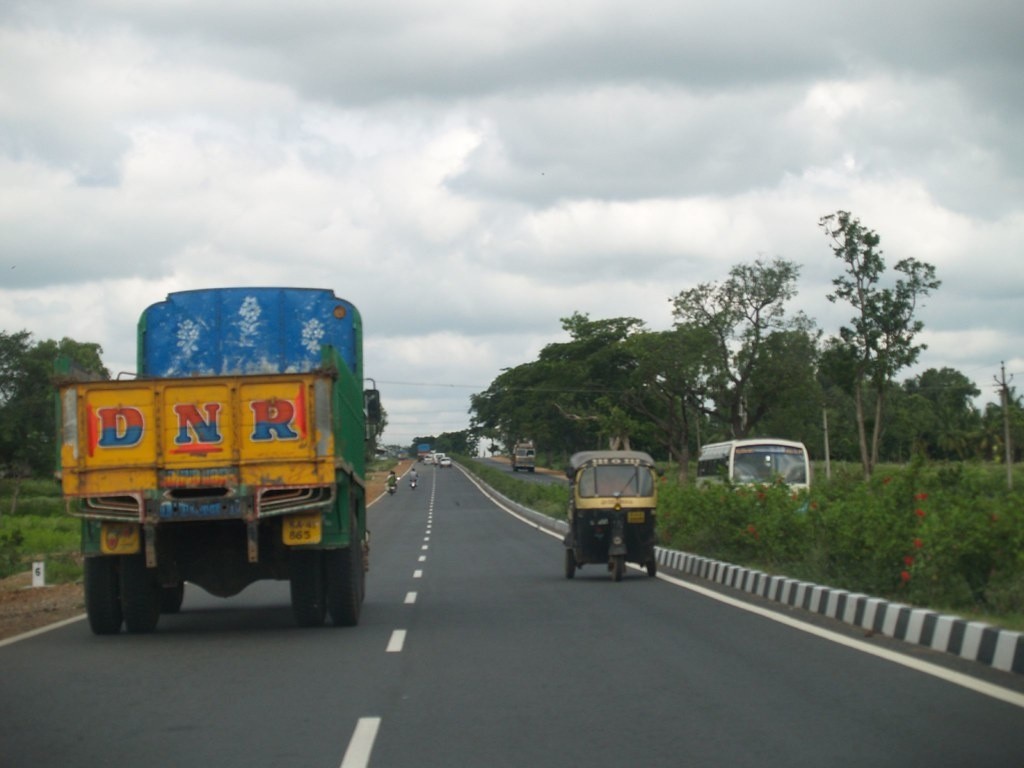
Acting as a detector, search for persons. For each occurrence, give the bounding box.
[409,468,418,483]
[387,470,398,487]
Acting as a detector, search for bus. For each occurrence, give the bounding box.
[510,443,537,474]
[696,434,809,512]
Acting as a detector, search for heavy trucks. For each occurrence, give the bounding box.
[53,282,386,636]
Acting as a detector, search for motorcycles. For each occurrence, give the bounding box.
[384,477,402,495]
[560,445,661,581]
[408,477,418,489]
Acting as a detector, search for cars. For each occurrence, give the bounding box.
[423,452,452,467]
